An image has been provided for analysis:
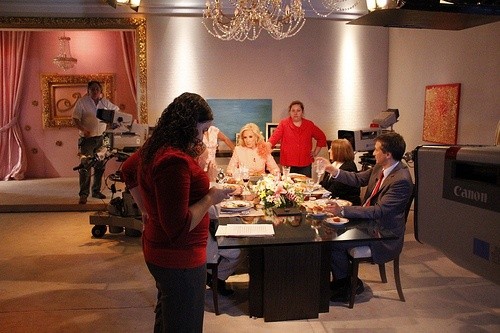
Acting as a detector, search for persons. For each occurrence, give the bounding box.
[315,132,413,303]
[120,93,237,333]
[318,138,362,206]
[226,122,281,174]
[71,79,120,204]
[266,101,329,178]
[195,126,240,296]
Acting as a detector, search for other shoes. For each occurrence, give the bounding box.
[330,284,373,304]
[92,191,106,199]
[209,278,240,299]
[329,282,344,292]
[78,196,87,204]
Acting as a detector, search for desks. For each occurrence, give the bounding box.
[215,173,400,322]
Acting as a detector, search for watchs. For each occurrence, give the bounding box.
[340,206,345,217]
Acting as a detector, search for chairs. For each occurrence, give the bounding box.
[344,183,417,310]
[207,256,223,315]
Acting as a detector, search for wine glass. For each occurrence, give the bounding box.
[313,160,326,187]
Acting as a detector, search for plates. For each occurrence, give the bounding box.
[219,199,252,211]
[324,217,349,225]
[316,198,352,208]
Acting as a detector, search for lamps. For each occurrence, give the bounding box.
[201,0,307,43]
[52,29,78,71]
[308,0,406,17]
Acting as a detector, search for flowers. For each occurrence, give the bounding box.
[253,172,301,208]
[256,205,295,226]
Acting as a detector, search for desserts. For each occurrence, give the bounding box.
[333,217,340,222]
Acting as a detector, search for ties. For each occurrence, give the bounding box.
[365,170,385,207]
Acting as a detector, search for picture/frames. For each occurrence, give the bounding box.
[235,133,241,146]
[40,72,119,130]
[266,123,280,151]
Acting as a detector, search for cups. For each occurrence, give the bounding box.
[282,166,290,175]
